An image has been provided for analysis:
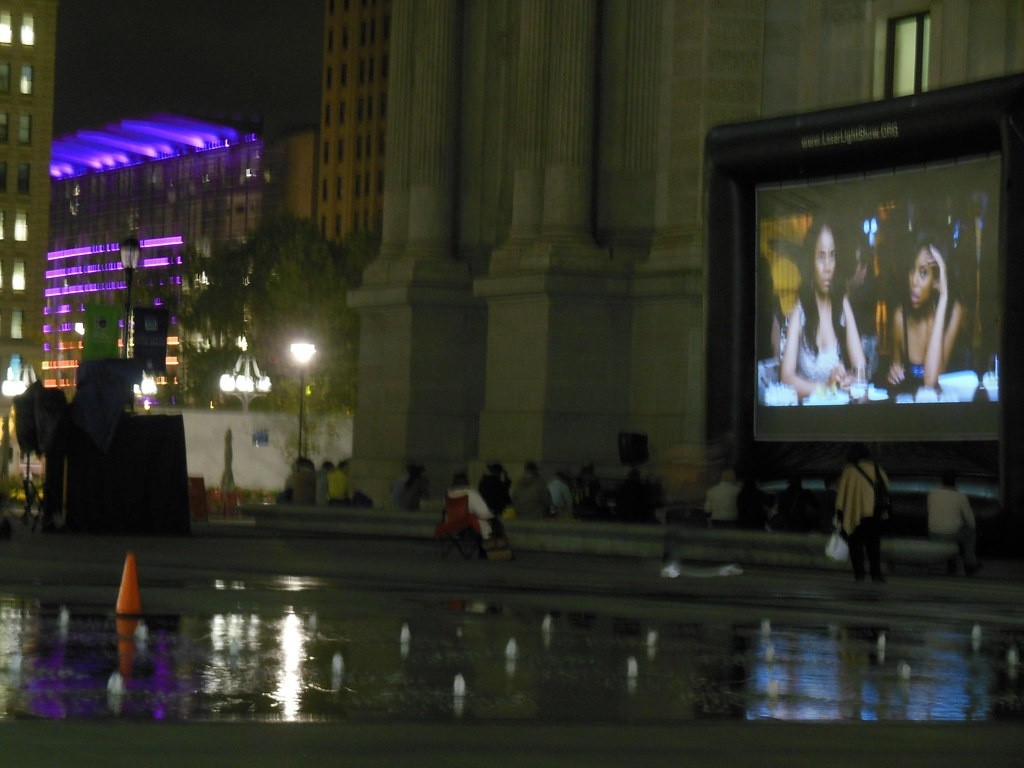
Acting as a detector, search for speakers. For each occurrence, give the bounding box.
[618,432,649,465]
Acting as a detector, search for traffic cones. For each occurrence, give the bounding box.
[107,549,151,621]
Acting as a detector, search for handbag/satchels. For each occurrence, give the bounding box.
[826,526,849,564]
[873,461,888,518]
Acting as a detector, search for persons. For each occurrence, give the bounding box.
[285,457,353,503]
[479,461,603,519]
[779,218,867,400]
[834,443,890,584]
[703,469,777,527]
[433,469,514,541]
[926,472,977,552]
[612,451,663,522]
[886,234,963,388]
[390,464,431,513]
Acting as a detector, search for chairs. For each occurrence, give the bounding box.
[441,490,508,559]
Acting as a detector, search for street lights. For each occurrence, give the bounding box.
[287,338,320,471]
[116,231,145,365]
